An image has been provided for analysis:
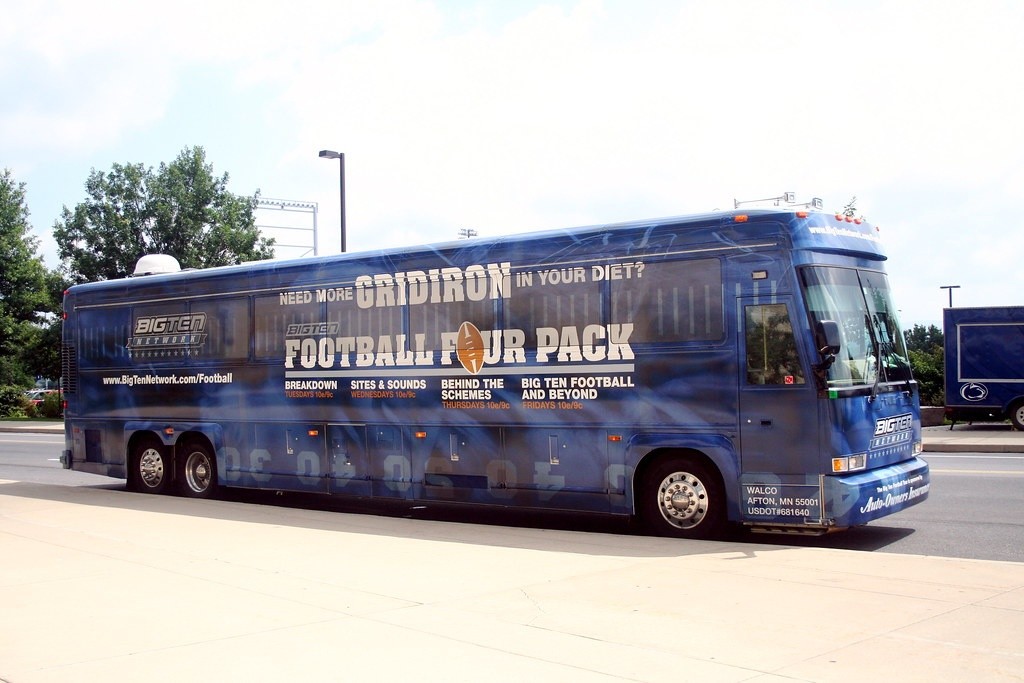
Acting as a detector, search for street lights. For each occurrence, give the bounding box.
[319,150,347,252]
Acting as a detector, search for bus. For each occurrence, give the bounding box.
[59,193,928,540]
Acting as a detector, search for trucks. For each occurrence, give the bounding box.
[942,306,1024,432]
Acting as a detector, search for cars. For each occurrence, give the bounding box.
[25,390,54,409]
